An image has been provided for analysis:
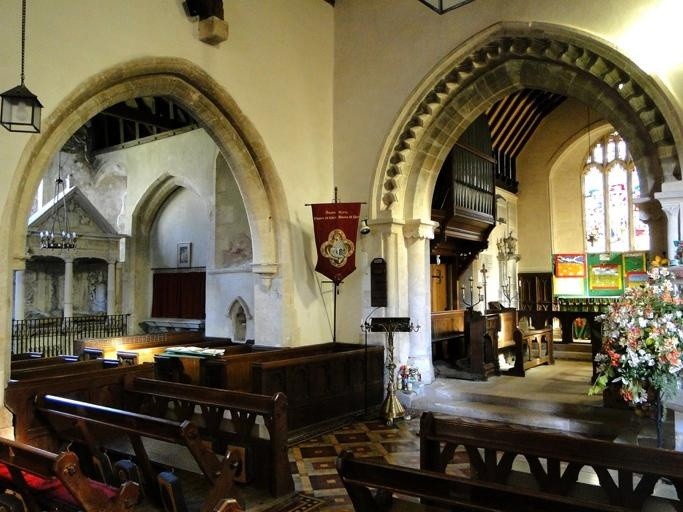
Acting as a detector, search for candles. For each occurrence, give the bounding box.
[460,263,485,300]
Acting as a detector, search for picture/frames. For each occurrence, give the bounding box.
[177,243,192,268]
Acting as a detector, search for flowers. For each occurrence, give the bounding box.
[585,255,683,423]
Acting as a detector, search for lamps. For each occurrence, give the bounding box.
[0,0,44,133]
[40,152,80,251]
[360,221,370,234]
[418,0,475,15]
[586,105,597,246]
[497,229,522,261]
